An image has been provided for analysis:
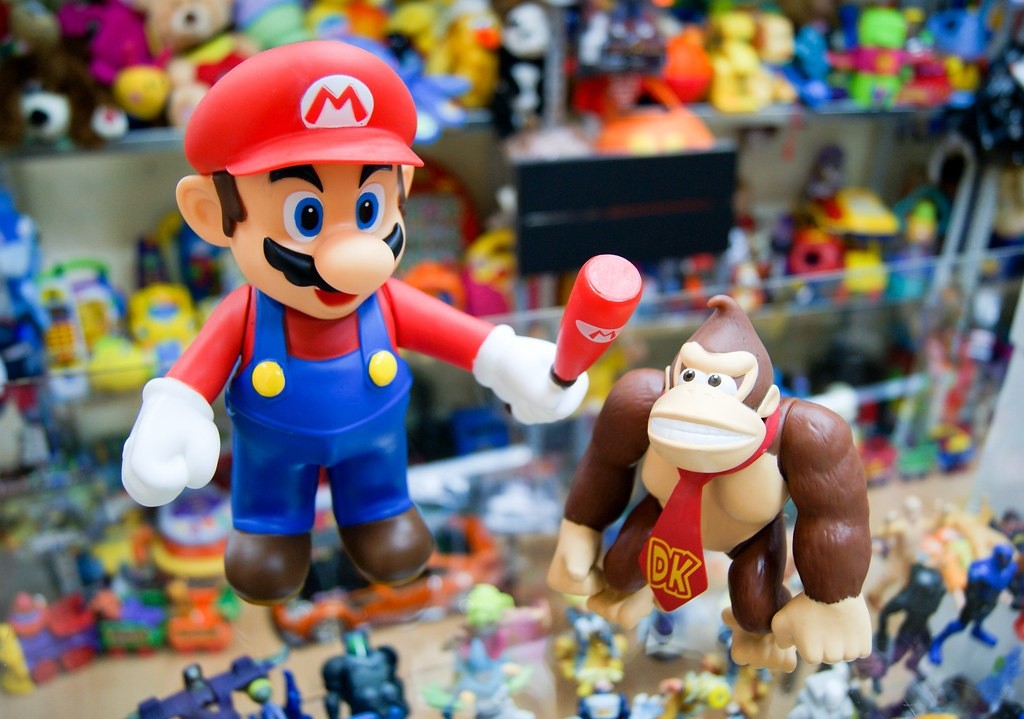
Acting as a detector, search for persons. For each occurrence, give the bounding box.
[873,544,946,693]
[122,42,588,604]
[930,544,1018,664]
[244,585,859,719]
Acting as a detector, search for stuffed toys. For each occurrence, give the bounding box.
[0,0,549,154]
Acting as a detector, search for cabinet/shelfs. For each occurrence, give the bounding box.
[0,90,1024,718]
